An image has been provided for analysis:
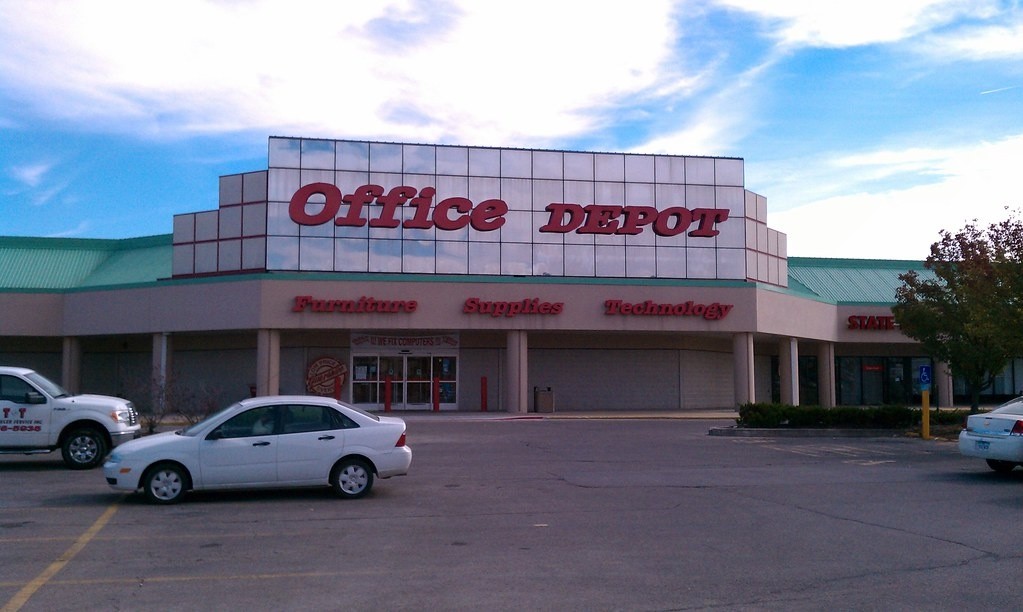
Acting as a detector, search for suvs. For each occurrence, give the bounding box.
[0,368,140,470]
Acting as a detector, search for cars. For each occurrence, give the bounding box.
[101,394,413,504]
[958,395,1023,474]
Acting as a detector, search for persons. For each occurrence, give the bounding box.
[246,408,276,436]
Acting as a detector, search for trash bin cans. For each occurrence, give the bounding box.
[533,385,553,413]
